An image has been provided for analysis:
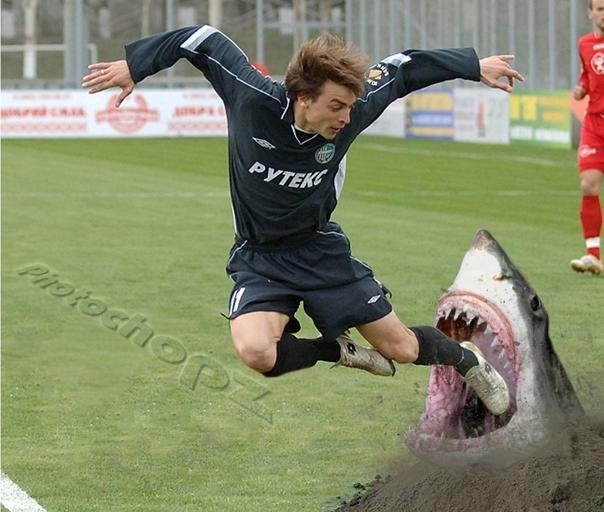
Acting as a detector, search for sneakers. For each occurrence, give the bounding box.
[337,328,396,375]
[571,254,602,275]
[456,341,509,415]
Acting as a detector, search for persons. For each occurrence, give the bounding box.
[80,20,526,417]
[566,0,603,277]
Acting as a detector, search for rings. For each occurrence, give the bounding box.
[99,69,104,75]
